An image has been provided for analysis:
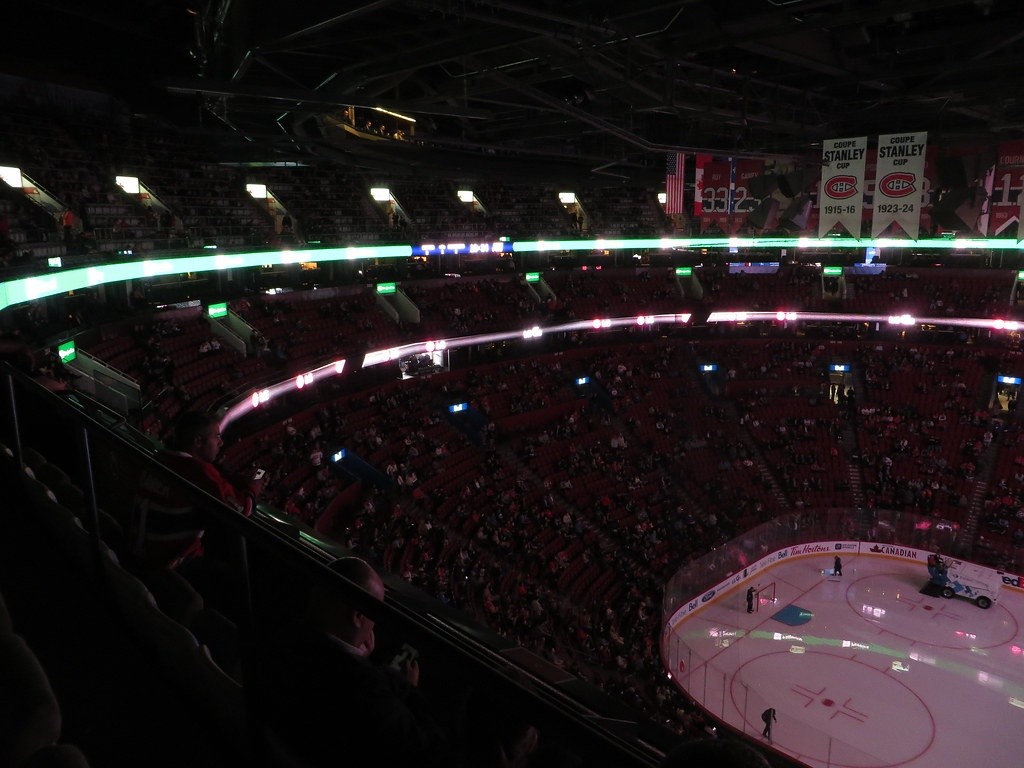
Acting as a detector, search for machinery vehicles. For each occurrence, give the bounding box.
[918,552,1002,609]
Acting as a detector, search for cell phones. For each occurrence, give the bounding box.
[387,641,418,678]
[253,466,268,480]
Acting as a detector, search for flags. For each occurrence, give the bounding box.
[664,151,684,214]
[693,153,712,216]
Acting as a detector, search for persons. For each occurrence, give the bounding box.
[760,708,777,740]
[0,150,1024,768]
[833,554,843,576]
[746,587,756,614]
